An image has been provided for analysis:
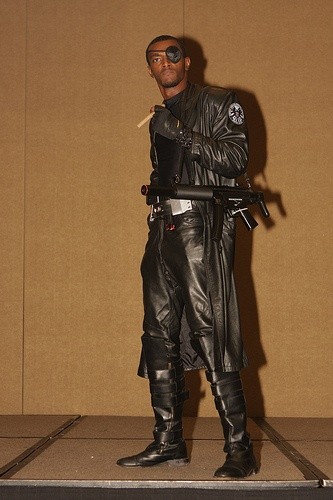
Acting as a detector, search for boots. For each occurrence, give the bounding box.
[117,367,191,468]
[199,371,258,478]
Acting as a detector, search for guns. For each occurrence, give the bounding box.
[140,183,271,242]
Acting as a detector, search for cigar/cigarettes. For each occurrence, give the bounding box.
[137,104,165,128]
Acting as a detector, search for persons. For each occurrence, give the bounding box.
[117,35,257,480]
